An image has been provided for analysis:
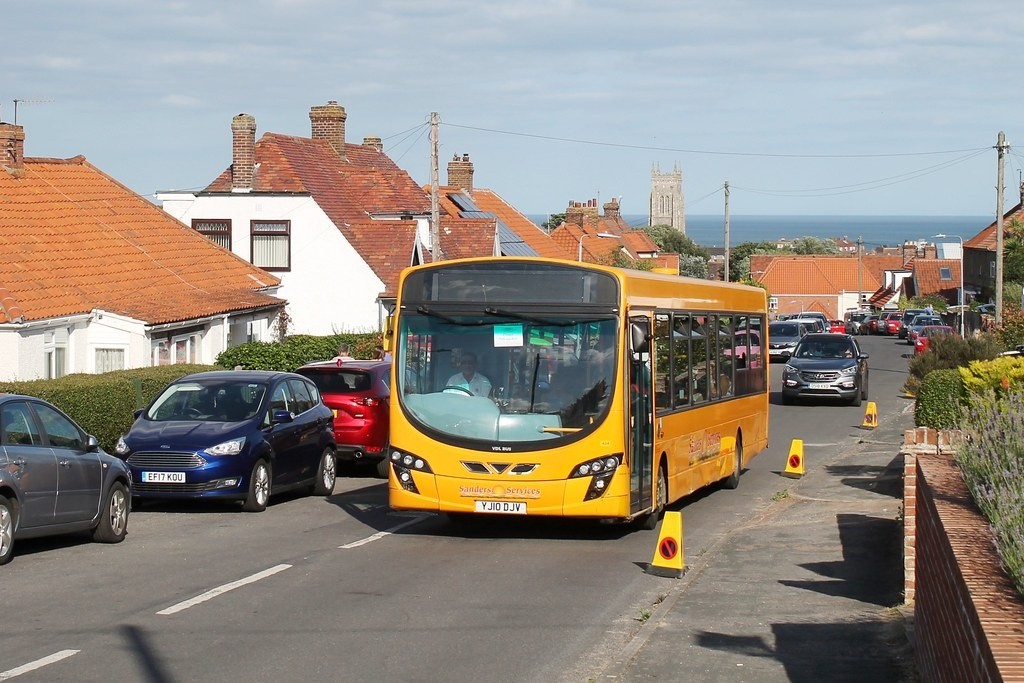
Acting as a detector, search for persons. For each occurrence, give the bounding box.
[708,359,733,400]
[443,352,492,397]
[330,343,393,363]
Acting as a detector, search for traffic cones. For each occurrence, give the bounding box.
[646,511,690,580]
[859,402,878,431]
[903,375,916,399]
[782,438,808,481]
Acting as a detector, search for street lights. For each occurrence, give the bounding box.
[930,233,965,340]
[579,233,622,261]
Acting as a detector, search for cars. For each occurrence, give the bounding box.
[112,370,338,512]
[781,310,888,334]
[782,331,870,407]
[0,393,133,565]
[722,329,761,368]
[911,325,962,356]
[906,314,948,344]
[766,320,808,364]
[286,359,424,479]
[884,312,904,336]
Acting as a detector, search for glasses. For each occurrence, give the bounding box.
[460,360,472,365]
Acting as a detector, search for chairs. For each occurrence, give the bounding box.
[183,386,227,420]
[239,386,282,423]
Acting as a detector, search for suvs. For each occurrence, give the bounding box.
[897,309,935,339]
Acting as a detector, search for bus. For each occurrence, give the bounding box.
[382,255,770,530]
